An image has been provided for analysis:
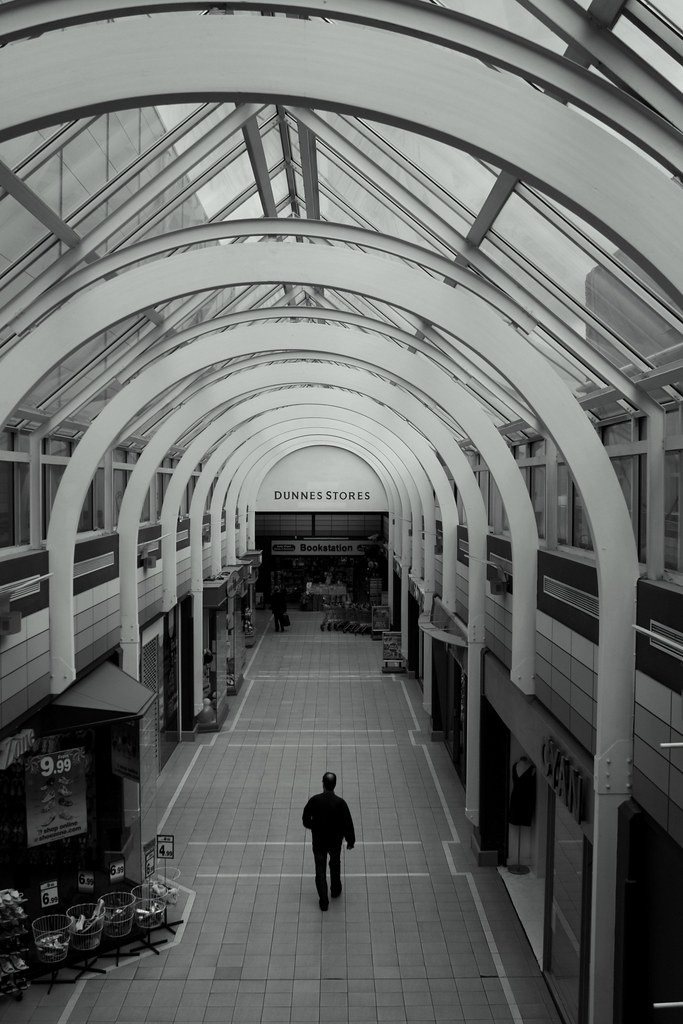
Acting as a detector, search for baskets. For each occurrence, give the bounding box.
[66,903,106,950]
[146,866,181,908]
[131,884,169,929]
[98,892,136,937]
[32,914,73,963]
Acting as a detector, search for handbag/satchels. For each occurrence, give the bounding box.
[282,615,290,626]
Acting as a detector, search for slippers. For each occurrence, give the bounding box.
[0,955,29,974]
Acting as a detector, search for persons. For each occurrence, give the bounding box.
[270,586,287,632]
[302,772,355,911]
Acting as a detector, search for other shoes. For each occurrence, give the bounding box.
[0,889,28,938]
[0,973,28,994]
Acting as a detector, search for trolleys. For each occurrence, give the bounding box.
[321,600,383,635]
